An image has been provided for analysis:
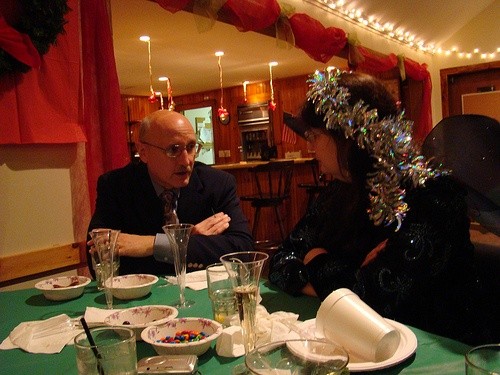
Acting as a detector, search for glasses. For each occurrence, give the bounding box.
[141,137,204,157]
[304,128,330,144]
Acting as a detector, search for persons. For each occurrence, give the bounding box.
[269,73,488,349]
[86,110,252,278]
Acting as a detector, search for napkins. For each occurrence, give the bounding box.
[0,312,92,354]
[164,265,238,291]
[216,306,297,359]
[81,305,122,328]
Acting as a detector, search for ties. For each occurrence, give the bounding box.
[162,188,180,226]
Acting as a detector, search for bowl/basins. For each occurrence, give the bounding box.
[141,316,223,357]
[101,273,160,300]
[104,305,179,341]
[35,275,91,300]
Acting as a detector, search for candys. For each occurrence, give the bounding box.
[155,330,208,344]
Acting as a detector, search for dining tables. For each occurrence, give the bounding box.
[0,264,500,375]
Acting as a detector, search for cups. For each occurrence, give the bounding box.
[244,338,350,375]
[316,287,400,364]
[74,326,138,375]
[206,262,240,329]
[90,243,120,290]
[463,343,500,375]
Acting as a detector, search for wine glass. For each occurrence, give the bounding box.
[88,230,121,309]
[219,251,269,375]
[162,224,194,309]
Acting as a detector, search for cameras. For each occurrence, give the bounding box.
[136,354,199,375]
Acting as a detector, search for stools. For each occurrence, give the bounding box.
[299,161,335,215]
[239,159,295,251]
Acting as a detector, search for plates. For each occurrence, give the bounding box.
[286,317,418,371]
